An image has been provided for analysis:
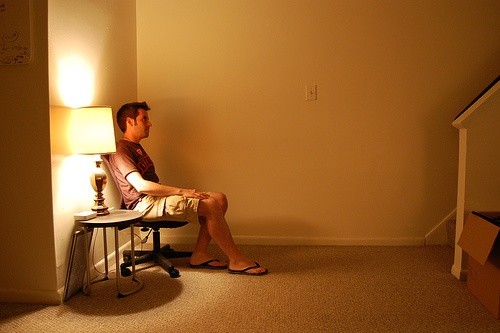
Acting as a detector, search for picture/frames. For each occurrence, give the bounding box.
[62,228,94,302]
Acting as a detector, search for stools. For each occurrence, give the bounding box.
[79,209,144,298]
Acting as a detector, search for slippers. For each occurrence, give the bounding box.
[188,258,228,271]
[227,261,269,276]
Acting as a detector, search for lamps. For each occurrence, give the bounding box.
[64,106,118,216]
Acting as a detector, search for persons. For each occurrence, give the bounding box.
[109,101,268,275]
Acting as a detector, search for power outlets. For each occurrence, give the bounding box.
[306,84,317,101]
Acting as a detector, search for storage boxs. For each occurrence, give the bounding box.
[457,210,500,320]
[73,211,97,220]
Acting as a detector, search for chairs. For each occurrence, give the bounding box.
[100,154,193,278]
